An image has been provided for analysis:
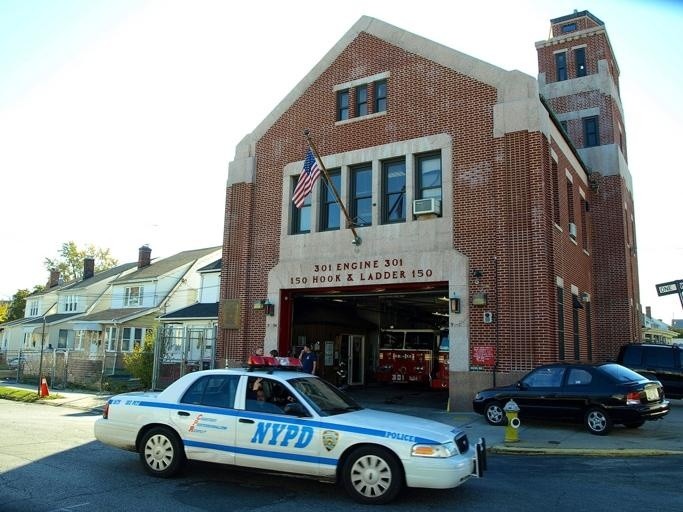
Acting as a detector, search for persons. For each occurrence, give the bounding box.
[251,377,272,413]
[254,345,264,357]
[270,350,279,358]
[297,344,316,374]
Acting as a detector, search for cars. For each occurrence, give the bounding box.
[94,355,486,505]
[473,362,672,435]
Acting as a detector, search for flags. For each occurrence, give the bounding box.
[291,129,326,211]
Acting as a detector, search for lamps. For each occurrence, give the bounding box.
[264,299,274,316]
[450,293,461,313]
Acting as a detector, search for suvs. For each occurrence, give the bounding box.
[617,343,683,400]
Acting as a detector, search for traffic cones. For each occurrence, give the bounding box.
[42,378,49,397]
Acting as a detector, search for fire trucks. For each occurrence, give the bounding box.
[376,327,449,389]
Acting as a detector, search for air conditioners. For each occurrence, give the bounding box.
[569,222,577,239]
[413,198,441,215]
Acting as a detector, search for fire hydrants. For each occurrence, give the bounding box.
[503,399,521,442]
